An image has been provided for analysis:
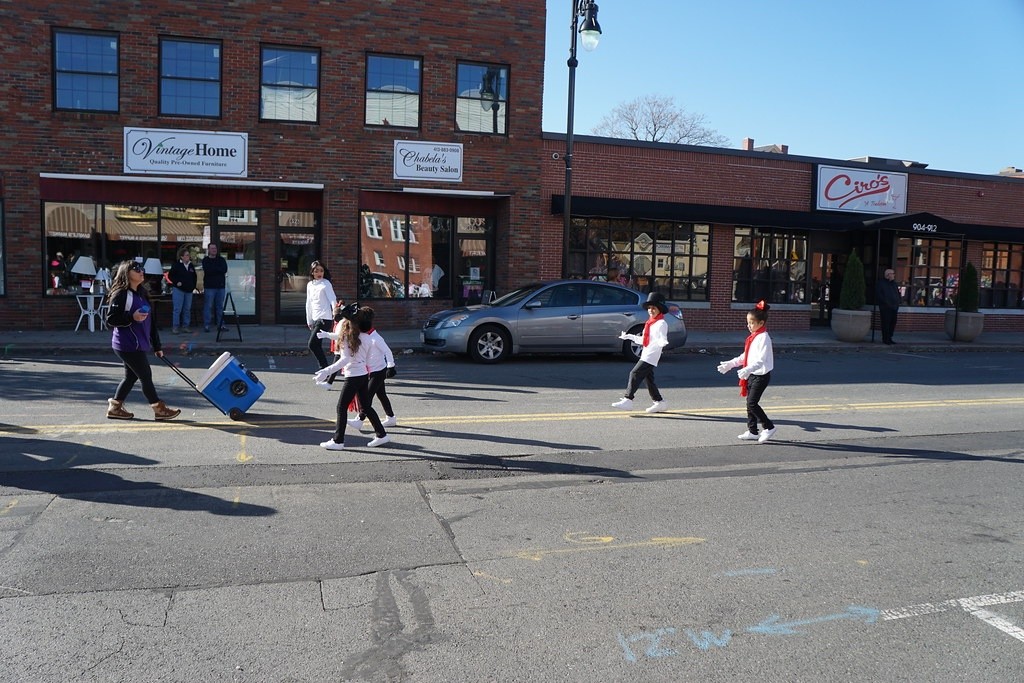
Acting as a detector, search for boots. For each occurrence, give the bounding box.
[151,400,181,421]
[106,397,134,420]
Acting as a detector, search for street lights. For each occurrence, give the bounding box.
[479,66,501,139]
[561,0,603,277]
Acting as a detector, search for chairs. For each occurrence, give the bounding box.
[553,288,588,306]
[100,305,117,332]
[599,295,632,305]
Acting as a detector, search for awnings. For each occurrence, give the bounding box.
[460,230,486,258]
[40,200,315,245]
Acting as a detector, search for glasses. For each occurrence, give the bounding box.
[129,266,146,274]
[888,273,895,275]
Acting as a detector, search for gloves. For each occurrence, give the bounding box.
[737,366,752,380]
[386,366,396,379]
[317,329,328,340]
[658,340,669,348]
[315,369,328,384]
[716,361,735,374]
[618,331,635,341]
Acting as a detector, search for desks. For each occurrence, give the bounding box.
[75,292,110,333]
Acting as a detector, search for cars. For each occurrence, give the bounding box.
[732,269,762,301]
[684,270,708,291]
[363,272,404,293]
[419,277,688,364]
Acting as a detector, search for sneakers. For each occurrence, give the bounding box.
[612,398,633,410]
[365,435,389,448]
[382,416,396,426]
[738,430,759,440]
[646,401,667,412]
[758,425,776,443]
[319,382,332,391]
[320,438,343,450]
[345,415,364,431]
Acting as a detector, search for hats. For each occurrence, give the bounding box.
[643,292,668,314]
[56,252,63,257]
[342,300,361,321]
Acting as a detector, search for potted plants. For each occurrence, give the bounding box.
[831,247,872,341]
[943,260,984,342]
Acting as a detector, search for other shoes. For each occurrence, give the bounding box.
[219,326,229,332]
[172,328,179,334]
[182,327,193,333]
[882,337,897,345]
[204,326,209,332]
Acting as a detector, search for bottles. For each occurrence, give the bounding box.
[138,306,150,315]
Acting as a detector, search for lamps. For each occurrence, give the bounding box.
[70,256,96,279]
[143,258,163,275]
[95,270,108,286]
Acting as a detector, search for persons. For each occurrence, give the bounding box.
[306,259,396,450]
[201,243,230,333]
[606,267,624,286]
[717,300,778,443]
[105,260,181,421]
[877,269,901,344]
[612,292,670,413]
[168,247,198,334]
